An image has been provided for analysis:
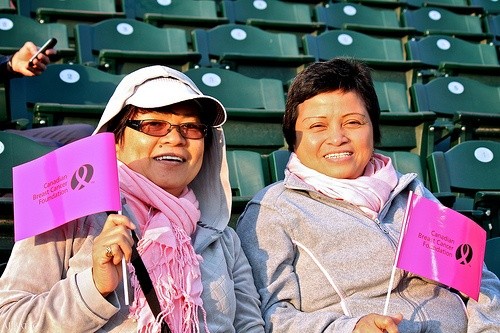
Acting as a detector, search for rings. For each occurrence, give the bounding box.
[106,245,112,259]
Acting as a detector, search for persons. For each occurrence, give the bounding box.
[1,65,265,333]
[236,58,500,332]
[0,41,93,144]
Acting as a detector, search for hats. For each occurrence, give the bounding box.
[124,77,227,128]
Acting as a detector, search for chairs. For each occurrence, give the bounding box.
[0,0,500,276]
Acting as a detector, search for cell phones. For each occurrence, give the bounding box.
[28,38,57,67]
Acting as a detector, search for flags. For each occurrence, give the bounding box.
[11,132,123,244]
[393,189,486,303]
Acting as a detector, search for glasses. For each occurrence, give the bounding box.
[125,119,209,140]
